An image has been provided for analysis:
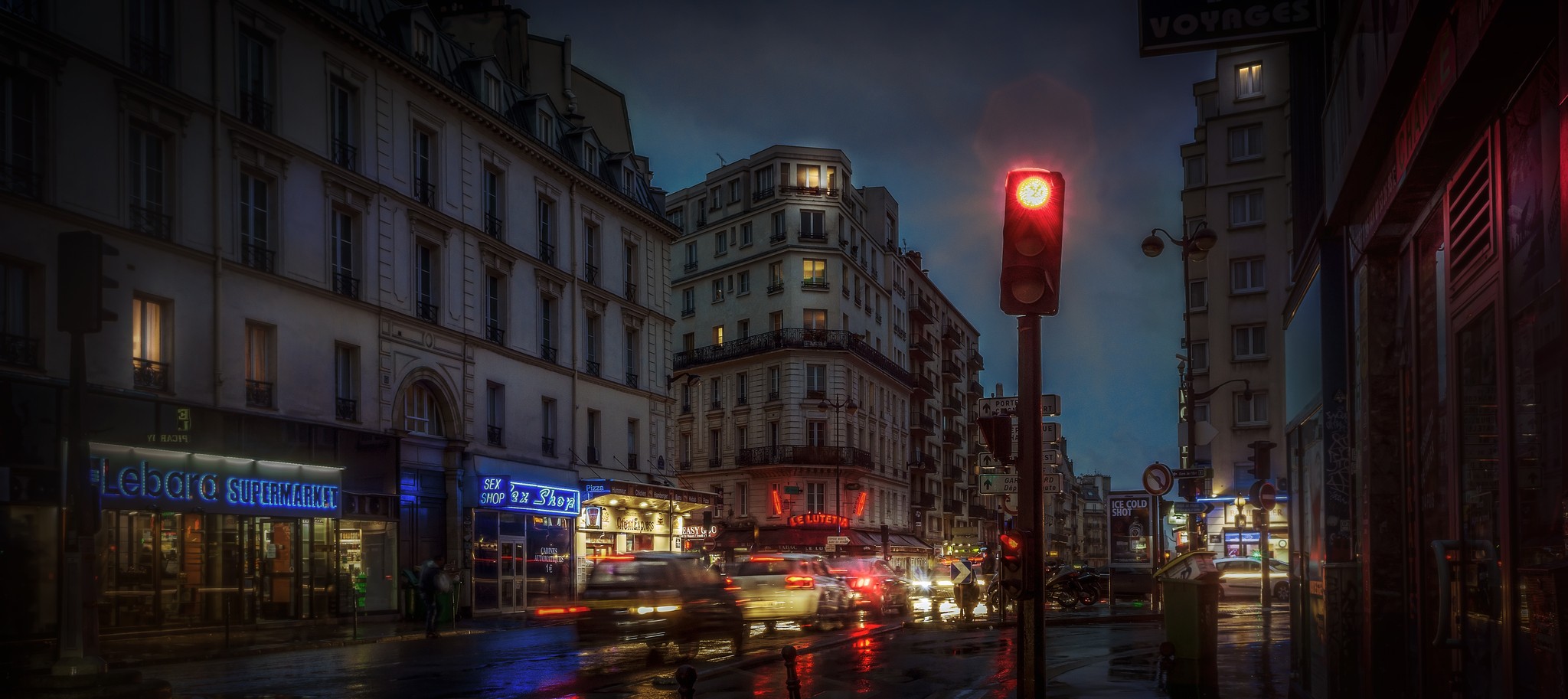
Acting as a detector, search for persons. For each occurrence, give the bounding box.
[981,545,995,605]
[418,547,452,638]
[707,558,721,574]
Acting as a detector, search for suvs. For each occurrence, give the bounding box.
[575,550,740,659]
[1213,557,1290,603]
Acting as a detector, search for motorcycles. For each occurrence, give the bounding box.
[988,552,1100,608]
[935,547,987,619]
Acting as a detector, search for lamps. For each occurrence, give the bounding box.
[922,269,929,274]
[667,373,701,390]
[1078,539,1084,543]
[906,461,925,471]
[1064,533,1072,537]
[1044,523,1052,527]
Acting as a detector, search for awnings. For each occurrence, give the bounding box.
[708,529,934,554]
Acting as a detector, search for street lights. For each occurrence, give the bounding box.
[818,392,858,559]
[1235,492,1247,557]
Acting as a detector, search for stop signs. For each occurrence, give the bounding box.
[1259,484,1278,511]
[703,537,716,550]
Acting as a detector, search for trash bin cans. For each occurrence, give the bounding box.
[1159,576,1226,661]
[402,568,462,622]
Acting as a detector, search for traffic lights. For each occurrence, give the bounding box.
[1246,441,1277,480]
[999,529,1025,600]
[999,169,1066,316]
[975,415,1012,462]
[1165,550,1170,560]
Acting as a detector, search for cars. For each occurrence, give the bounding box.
[1089,564,1162,602]
[717,554,911,633]
[924,563,984,599]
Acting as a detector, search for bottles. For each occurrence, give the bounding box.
[333,532,366,606]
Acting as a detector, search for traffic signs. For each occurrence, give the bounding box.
[978,393,1061,417]
[980,450,1062,466]
[980,474,1063,495]
[1174,501,1207,514]
[1171,469,1205,479]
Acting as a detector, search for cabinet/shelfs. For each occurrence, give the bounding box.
[334,529,363,587]
[138,528,177,567]
